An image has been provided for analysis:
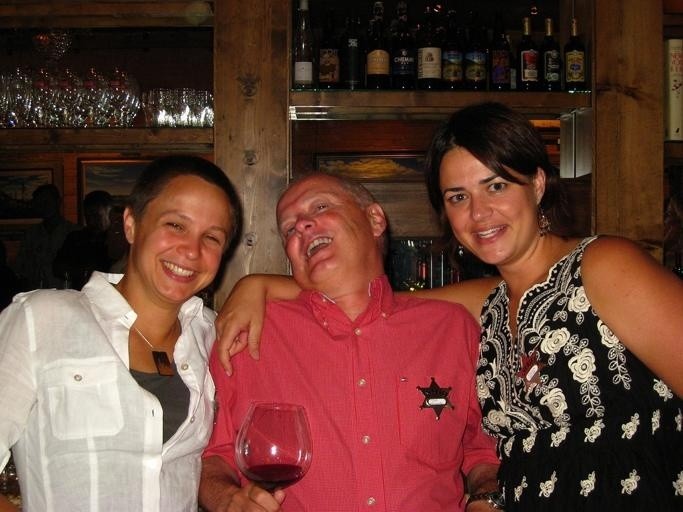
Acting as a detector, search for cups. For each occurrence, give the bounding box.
[142,88,214,127]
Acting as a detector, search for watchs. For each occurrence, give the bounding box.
[465,490,505,512]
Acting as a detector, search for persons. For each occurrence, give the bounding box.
[215,101,683,512]
[0,154,243,512]
[0,183,129,313]
[198,172,505,512]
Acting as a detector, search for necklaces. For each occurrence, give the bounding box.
[132,325,176,376]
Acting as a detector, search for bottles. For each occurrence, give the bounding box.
[402,241,430,293]
[293,1,588,91]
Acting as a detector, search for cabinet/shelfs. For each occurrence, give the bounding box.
[0,3,683,312]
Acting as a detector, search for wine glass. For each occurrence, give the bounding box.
[234,402,314,497]
